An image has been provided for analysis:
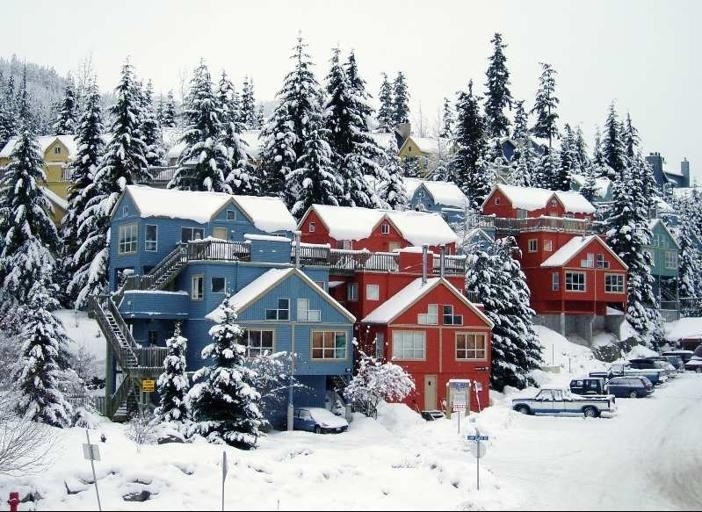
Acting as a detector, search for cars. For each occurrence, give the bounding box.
[568,346,699,398]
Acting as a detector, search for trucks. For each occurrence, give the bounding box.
[513,388,618,419]
